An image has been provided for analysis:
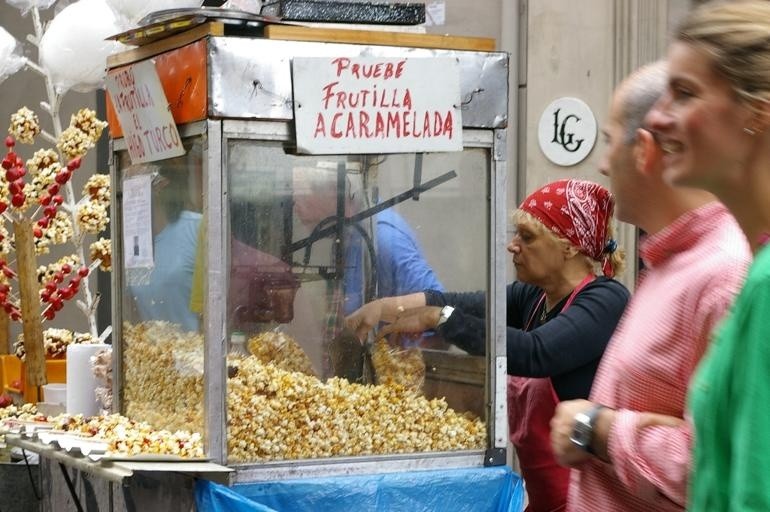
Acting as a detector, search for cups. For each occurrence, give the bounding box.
[39,381,67,405]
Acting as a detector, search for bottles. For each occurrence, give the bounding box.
[229,331,251,359]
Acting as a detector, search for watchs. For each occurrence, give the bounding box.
[570,403,617,464]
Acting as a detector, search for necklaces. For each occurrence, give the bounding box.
[540,301,555,324]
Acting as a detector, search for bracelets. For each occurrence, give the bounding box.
[438,306,454,324]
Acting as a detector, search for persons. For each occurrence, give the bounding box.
[344,175,633,511]
[540,56,751,511]
[642,0,769,512]
[292,156,444,388]
[132,159,205,336]
